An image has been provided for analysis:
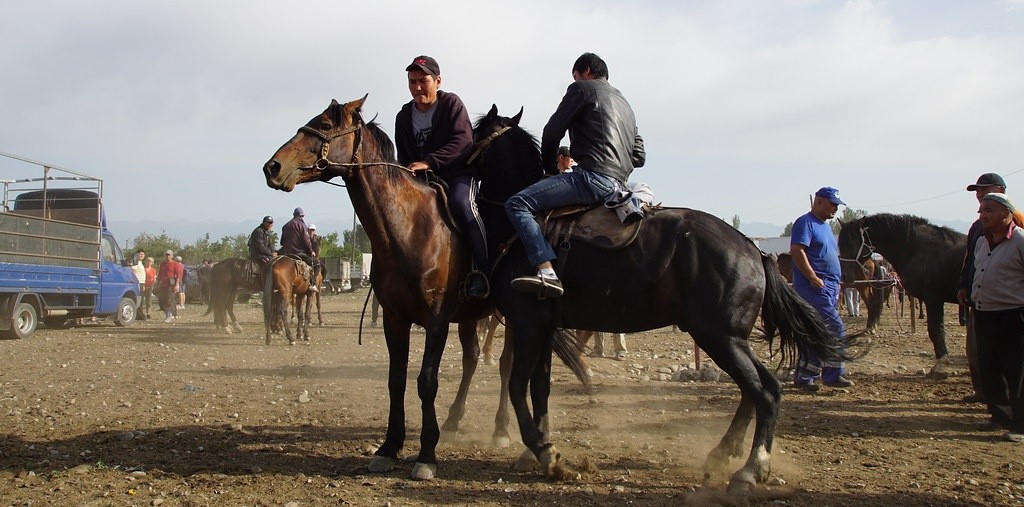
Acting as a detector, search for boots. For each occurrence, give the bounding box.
[164,312,172,323]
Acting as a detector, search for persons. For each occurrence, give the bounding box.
[790,188,854,392]
[845,260,888,318]
[505,53,646,297]
[307,224,318,242]
[248,216,277,285]
[958,173,1024,402]
[557,146,573,172]
[588,331,629,361]
[280,208,319,292]
[973,193,1024,441]
[130,249,215,323]
[395,56,488,295]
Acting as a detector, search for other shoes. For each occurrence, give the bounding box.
[848,314,860,318]
[175,305,185,310]
[823,376,854,387]
[793,380,819,392]
[147,313,151,319]
[309,285,319,293]
[510,273,564,298]
[617,353,626,361]
[587,352,605,358]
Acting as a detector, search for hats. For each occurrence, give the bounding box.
[308,223,316,231]
[816,187,846,206]
[175,256,183,262]
[560,145,571,154]
[293,208,303,218]
[984,192,1015,215]
[967,173,1007,191]
[166,250,173,256]
[406,55,440,77]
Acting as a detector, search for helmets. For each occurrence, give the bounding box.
[263,216,273,223]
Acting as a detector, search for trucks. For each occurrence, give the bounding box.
[0,151,143,339]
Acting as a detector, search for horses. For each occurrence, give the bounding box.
[837,212,970,380]
[262,93,593,481]
[201,258,265,336]
[465,101,845,495]
[264,234,328,346]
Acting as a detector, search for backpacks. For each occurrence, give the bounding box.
[183,267,191,283]
